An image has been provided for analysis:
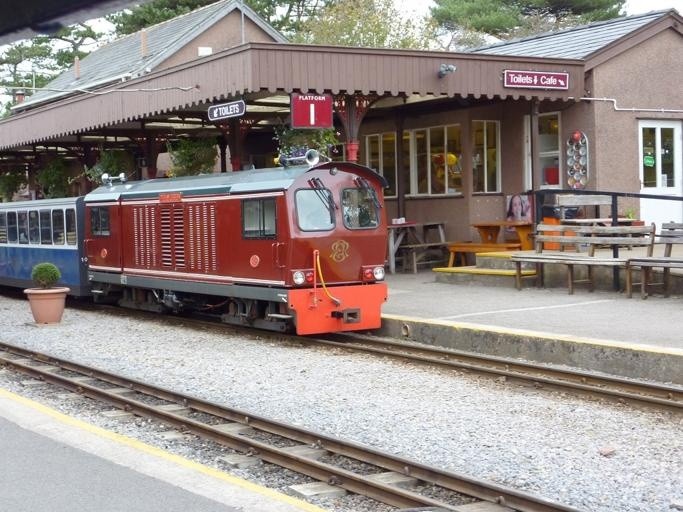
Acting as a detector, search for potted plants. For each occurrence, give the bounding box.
[79,166,95,195]
[67,174,78,198]
[23,262,71,324]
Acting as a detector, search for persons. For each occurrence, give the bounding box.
[507,194,527,230]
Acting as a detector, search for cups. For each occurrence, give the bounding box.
[392,217,405,225]
[544,167,558,185]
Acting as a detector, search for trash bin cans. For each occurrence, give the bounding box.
[542,204,579,251]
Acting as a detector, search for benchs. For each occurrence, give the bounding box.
[443,241,521,268]
[398,239,472,275]
[629,221,683,299]
[511,221,656,299]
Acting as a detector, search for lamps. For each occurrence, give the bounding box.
[439,63,457,79]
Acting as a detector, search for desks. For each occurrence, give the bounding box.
[472,220,534,250]
[384,219,448,275]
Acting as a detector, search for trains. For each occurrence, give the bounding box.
[0,149,393,337]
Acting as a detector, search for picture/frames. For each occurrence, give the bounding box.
[474,129,488,146]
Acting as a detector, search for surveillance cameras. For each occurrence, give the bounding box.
[448,64,457,72]
